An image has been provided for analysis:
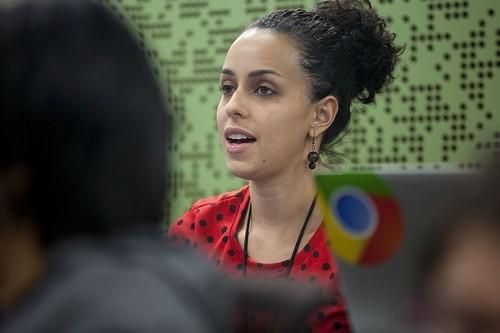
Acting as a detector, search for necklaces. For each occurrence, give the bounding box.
[239,186,319,333]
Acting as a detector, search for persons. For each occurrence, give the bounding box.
[162,0,406,333]
[0,0,232,333]
[412,151,500,333]
